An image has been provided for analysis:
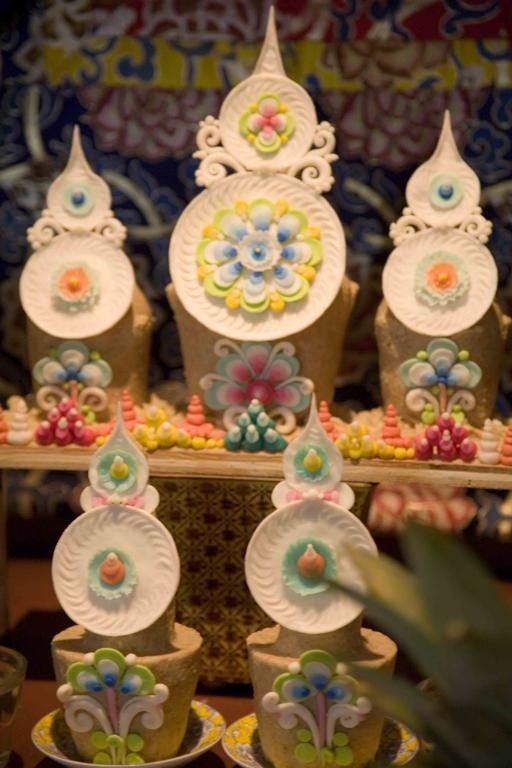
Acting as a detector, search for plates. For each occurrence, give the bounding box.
[0,404,512,768]
